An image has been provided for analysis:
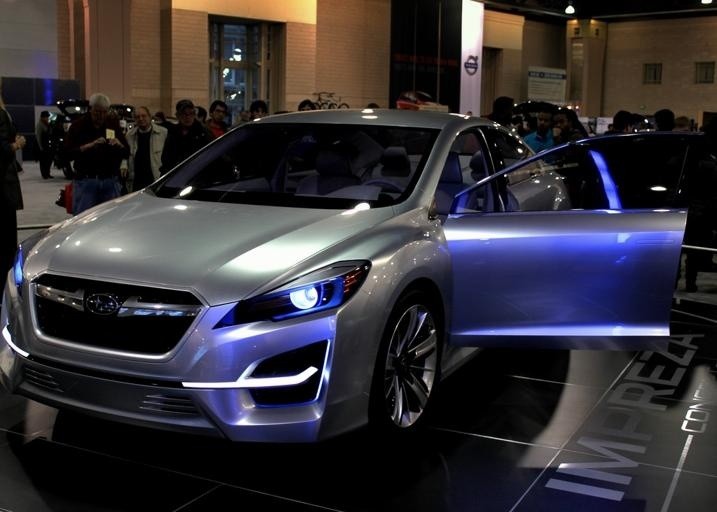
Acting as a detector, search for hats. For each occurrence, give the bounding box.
[176,100,194,114]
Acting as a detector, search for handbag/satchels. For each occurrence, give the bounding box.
[65,184,73,214]
[56,190,65,208]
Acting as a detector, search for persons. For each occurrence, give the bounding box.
[298,98,380,112]
[1,107,26,296]
[68,94,269,215]
[37,111,54,178]
[489,96,583,153]
[604,109,703,292]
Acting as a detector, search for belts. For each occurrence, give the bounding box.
[73,174,115,179]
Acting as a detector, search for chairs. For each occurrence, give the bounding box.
[415,152,476,213]
[468,150,485,186]
[369,144,417,189]
[291,149,361,196]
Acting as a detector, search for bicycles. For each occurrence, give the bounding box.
[311,92,338,110]
[320,93,349,110]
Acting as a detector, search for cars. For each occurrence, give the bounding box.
[1,107,704,445]
[45,98,179,177]
[480,100,590,211]
[395,90,442,110]
